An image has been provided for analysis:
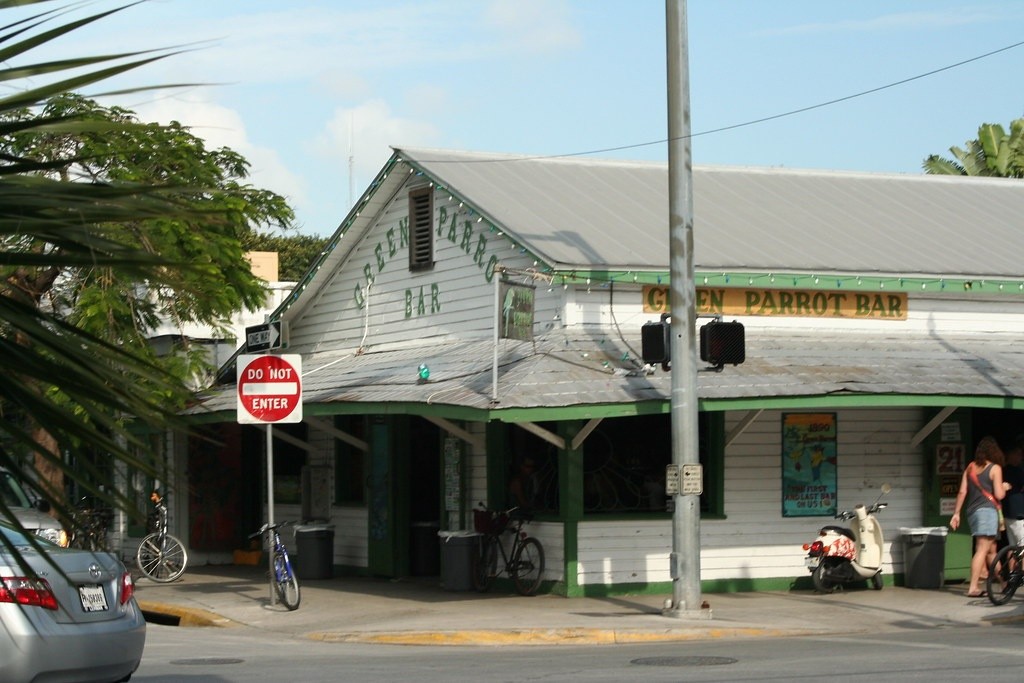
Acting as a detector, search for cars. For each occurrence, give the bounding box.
[0,518,146,683]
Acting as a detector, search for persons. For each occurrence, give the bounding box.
[145,490,167,579]
[949,436,1024,597]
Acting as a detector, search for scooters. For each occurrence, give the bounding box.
[802,483,893,593]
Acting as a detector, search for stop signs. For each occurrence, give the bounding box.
[239,356,302,422]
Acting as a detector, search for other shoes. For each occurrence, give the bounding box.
[963,588,987,597]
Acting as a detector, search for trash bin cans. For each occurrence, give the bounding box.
[440,531,483,592]
[294,523,335,579]
[901,525,947,588]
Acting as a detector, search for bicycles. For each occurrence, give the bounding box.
[986,543,1024,606]
[247,521,301,610]
[473,502,547,598]
[136,479,187,583]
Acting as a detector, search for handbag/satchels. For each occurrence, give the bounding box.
[995,502,1005,531]
